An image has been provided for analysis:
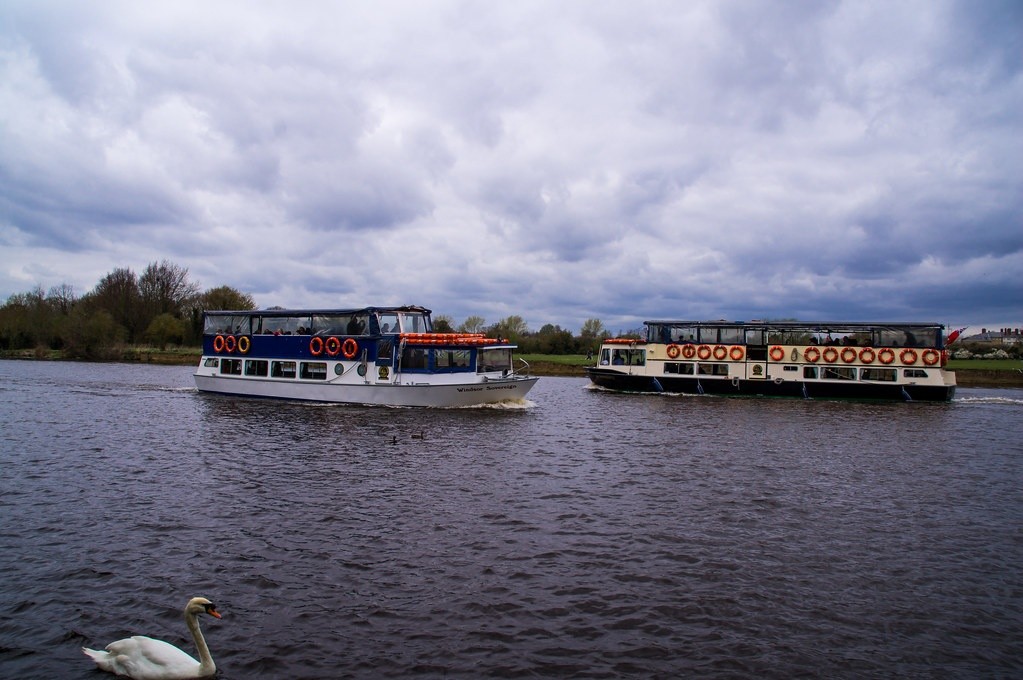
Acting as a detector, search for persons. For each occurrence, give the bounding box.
[687,335,696,342]
[614,349,642,365]
[214,313,400,336]
[678,336,686,342]
[586,347,593,360]
[808,329,927,347]
[221,359,308,379]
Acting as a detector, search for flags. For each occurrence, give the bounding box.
[947,326,968,346]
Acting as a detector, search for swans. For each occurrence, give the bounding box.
[81,597,223,680]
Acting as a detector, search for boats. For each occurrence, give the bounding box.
[192,305,541,410]
[581,317,958,407]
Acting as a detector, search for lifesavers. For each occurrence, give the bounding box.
[667,344,681,358]
[310,337,359,358]
[900,348,918,364]
[840,347,856,363]
[804,347,820,362]
[712,345,728,360]
[682,344,696,358]
[878,348,895,365]
[770,346,785,361]
[859,347,875,363]
[214,335,250,353]
[730,346,744,360]
[823,347,838,362]
[697,345,711,359]
[921,348,948,366]
